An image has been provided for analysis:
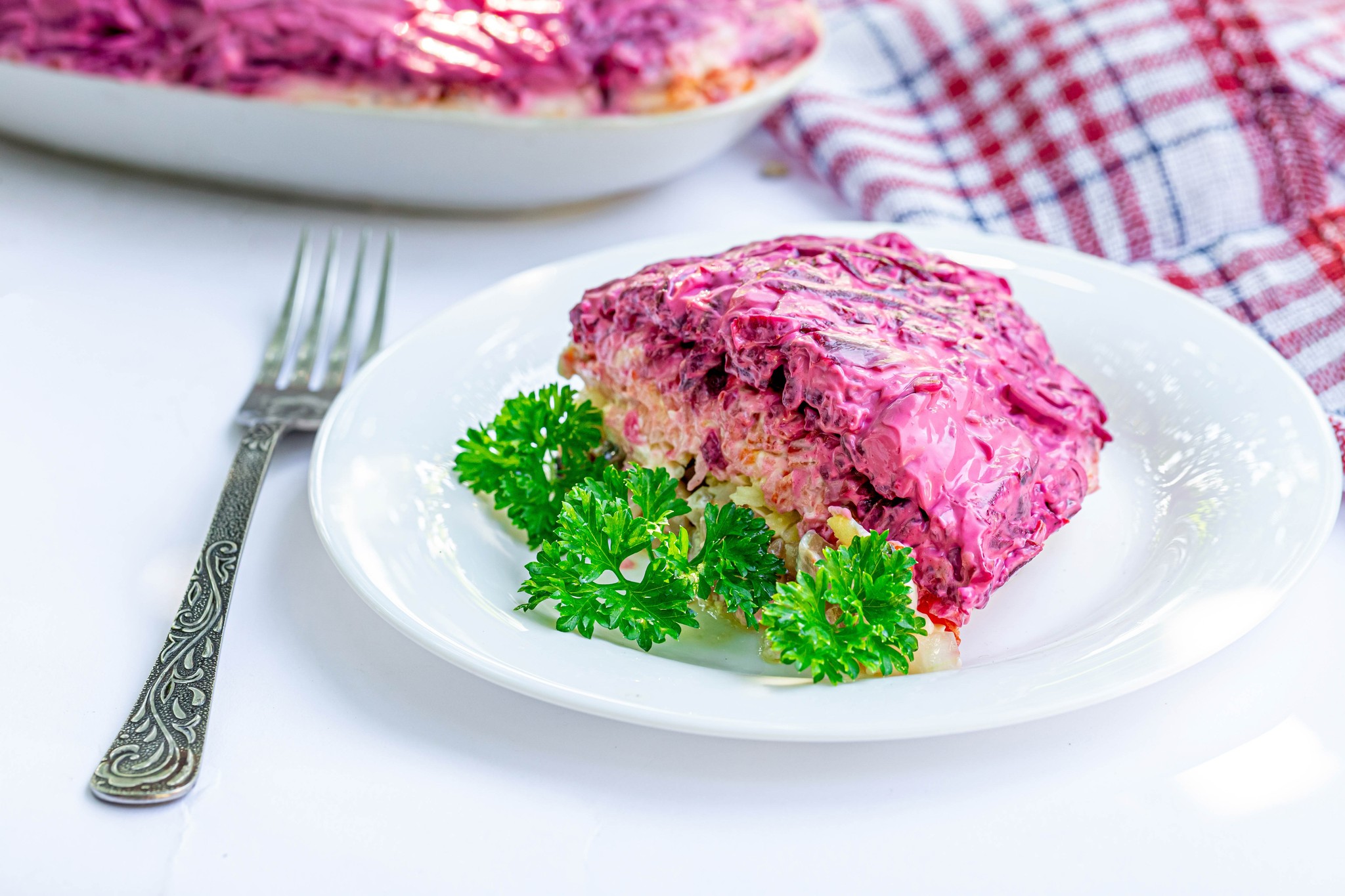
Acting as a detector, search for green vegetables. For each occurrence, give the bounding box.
[447,386,926,681]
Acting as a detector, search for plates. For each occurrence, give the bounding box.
[307,220,1345,745]
[0,0,830,210]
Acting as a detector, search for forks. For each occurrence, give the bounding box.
[84,227,395,805]
